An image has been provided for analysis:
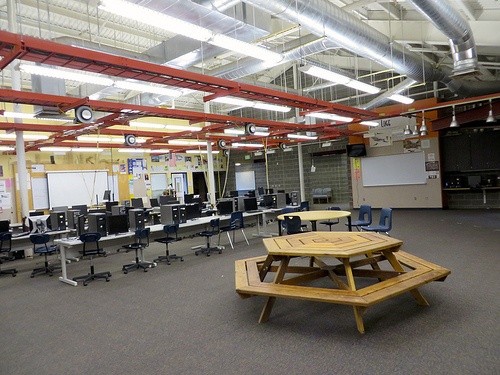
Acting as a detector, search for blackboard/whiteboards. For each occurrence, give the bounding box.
[361,150,427,187]
[235,170,255,190]
[32,175,119,211]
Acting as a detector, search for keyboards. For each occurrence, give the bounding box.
[246,211,263,213]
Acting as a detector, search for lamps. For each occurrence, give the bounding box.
[0,0,429,157]
[403,124,413,135]
[450,105,459,127]
[486,110,496,122]
[412,123,419,135]
[419,110,428,136]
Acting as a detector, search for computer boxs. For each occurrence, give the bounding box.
[290,191,300,205]
[112,205,126,214]
[160,204,181,226]
[179,205,187,223]
[66,210,81,229]
[262,195,278,209]
[88,213,106,237]
[51,211,67,230]
[129,209,145,233]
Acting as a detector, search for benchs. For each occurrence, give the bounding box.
[234,250,452,307]
[312,188,332,203]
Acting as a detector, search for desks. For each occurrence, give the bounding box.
[10,203,429,334]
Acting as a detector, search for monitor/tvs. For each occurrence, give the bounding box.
[274,193,286,207]
[72,205,87,217]
[29,211,44,217]
[89,190,207,213]
[106,215,128,235]
[218,202,232,216]
[230,187,285,198]
[52,206,69,220]
[29,214,51,233]
[0,221,10,233]
[185,204,199,220]
[244,197,258,211]
[346,143,366,156]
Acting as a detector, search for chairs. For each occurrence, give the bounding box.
[345,205,372,231]
[318,207,340,231]
[361,207,392,236]
[0,187,310,287]
